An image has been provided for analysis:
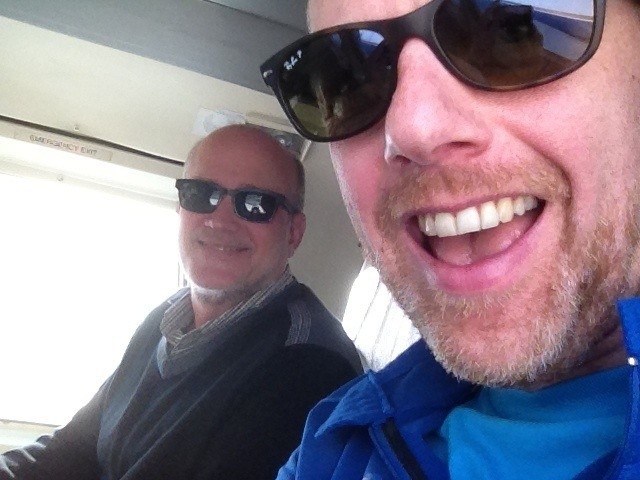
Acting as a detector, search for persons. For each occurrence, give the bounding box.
[258,0,640,479]
[0,122,366,480]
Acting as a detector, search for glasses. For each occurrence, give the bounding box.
[174,178,298,224]
[258,0,611,143]
